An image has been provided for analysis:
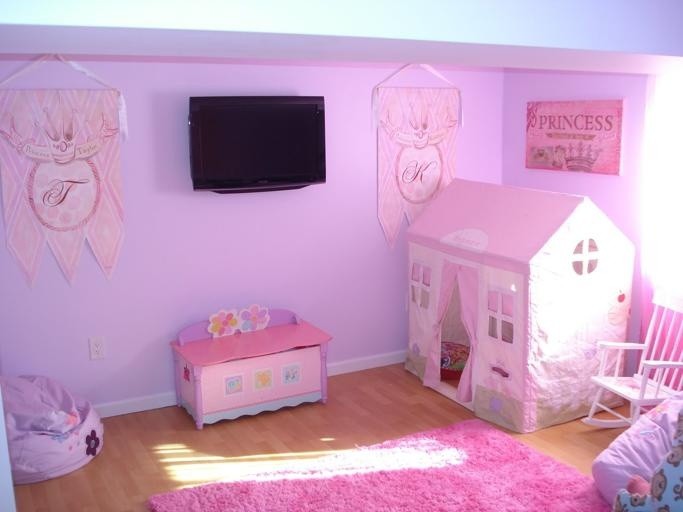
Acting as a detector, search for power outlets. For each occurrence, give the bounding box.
[89,336,106,360]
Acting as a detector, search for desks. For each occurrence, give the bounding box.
[169,306,332,431]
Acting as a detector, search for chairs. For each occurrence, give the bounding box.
[581,295,683,428]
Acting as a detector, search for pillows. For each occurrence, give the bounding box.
[440,341,470,380]
[591,390,682,507]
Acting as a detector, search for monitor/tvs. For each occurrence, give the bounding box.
[188,95,325,195]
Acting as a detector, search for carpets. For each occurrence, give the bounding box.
[143,418,612,512]
